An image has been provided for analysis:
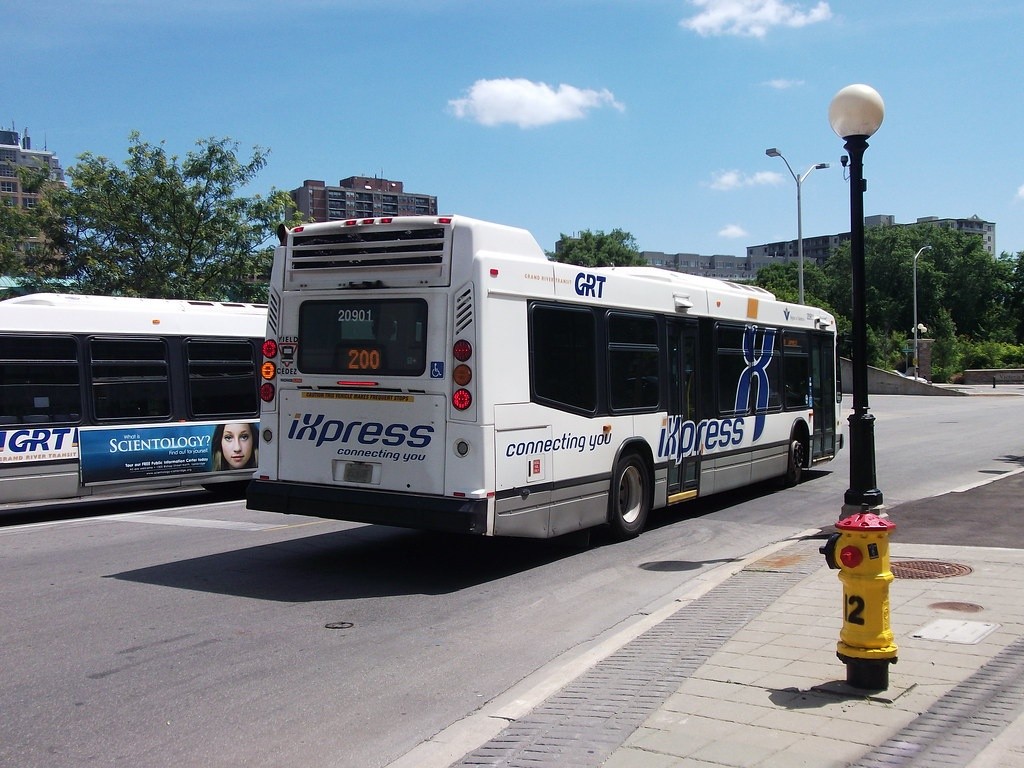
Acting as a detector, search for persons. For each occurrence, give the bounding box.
[210,424,259,471]
[371,314,396,341]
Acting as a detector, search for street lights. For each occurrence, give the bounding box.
[913,246,932,380]
[765,148,830,305]
[828,81,892,524]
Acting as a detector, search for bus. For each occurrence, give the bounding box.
[0,292,267,512]
[245,213,845,539]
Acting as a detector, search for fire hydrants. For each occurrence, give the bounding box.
[817,511,897,692]
